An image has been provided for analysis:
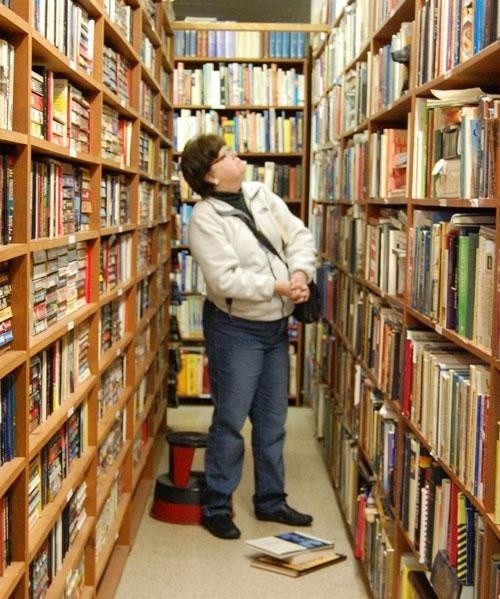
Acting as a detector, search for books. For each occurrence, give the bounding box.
[247,529,346,580]
[309,2,500,598]
[174,28,308,399]
[1,0,174,599]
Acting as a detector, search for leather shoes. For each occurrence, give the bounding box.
[203,515,240,539]
[254,503,312,526]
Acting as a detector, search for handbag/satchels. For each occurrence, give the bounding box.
[292,279,322,324]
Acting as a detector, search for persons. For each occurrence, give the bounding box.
[180,133,318,541]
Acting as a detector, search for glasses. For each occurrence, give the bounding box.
[211,150,230,166]
